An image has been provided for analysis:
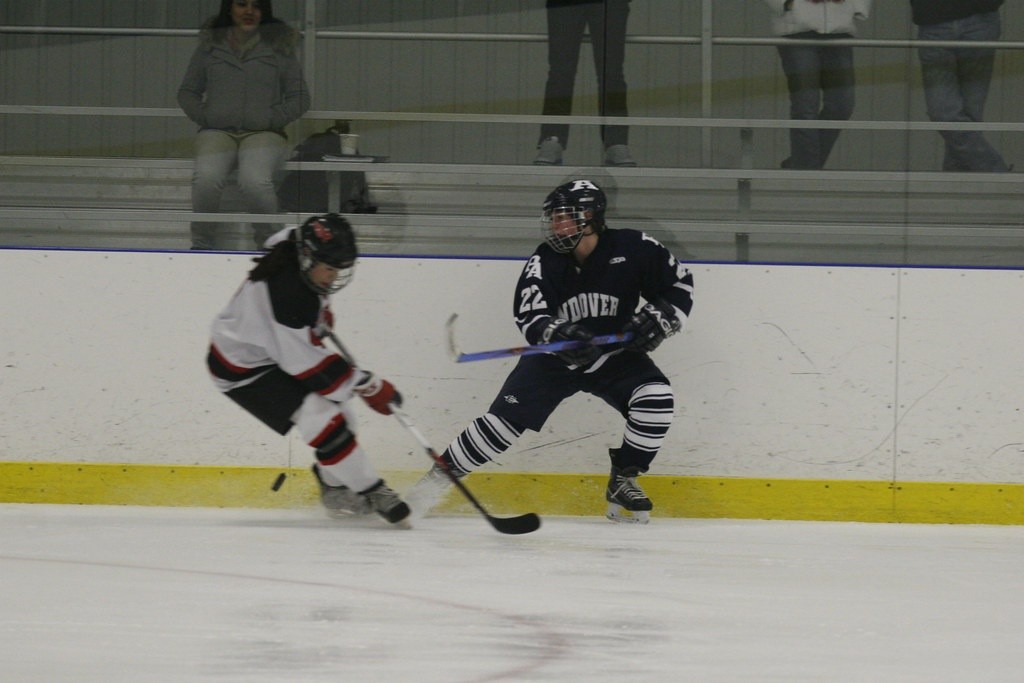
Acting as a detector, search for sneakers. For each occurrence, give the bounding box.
[606,448,653,524]
[356,478,412,526]
[392,468,452,529]
[311,463,375,514]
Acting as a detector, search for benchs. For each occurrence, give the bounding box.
[0,105,1024,266]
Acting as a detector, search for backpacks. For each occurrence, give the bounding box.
[277,119,370,211]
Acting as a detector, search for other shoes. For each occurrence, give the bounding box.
[600,144,638,167]
[531,135,564,167]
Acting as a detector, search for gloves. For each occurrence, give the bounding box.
[532,315,603,368]
[309,303,334,349]
[351,369,402,415]
[618,297,682,355]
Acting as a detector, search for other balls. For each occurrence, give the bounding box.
[271,472,286,491]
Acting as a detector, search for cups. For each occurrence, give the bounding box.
[339,134,360,154]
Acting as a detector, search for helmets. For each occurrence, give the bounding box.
[538,179,607,254]
[296,212,358,296]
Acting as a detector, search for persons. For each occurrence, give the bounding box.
[401,179,694,529]
[533,0,637,166]
[765,0,872,170]
[176,0,311,251]
[205,214,411,532]
[911,0,1013,171]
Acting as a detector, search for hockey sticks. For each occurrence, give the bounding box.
[323,326,542,536]
[441,310,633,365]
